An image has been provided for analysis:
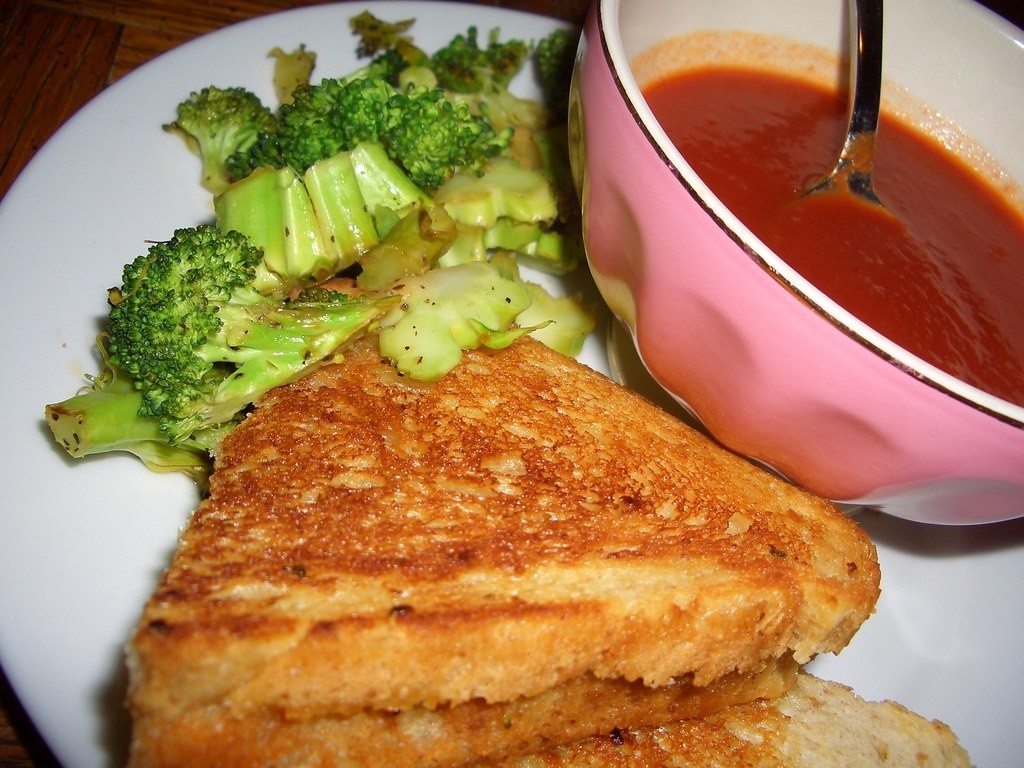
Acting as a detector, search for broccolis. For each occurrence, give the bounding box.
[40,9,605,496]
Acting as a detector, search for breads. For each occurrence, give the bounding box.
[121,276,974,768]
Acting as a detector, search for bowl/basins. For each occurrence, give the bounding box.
[565,0,1023,525]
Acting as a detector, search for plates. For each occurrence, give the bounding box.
[0,0,1024,768]
